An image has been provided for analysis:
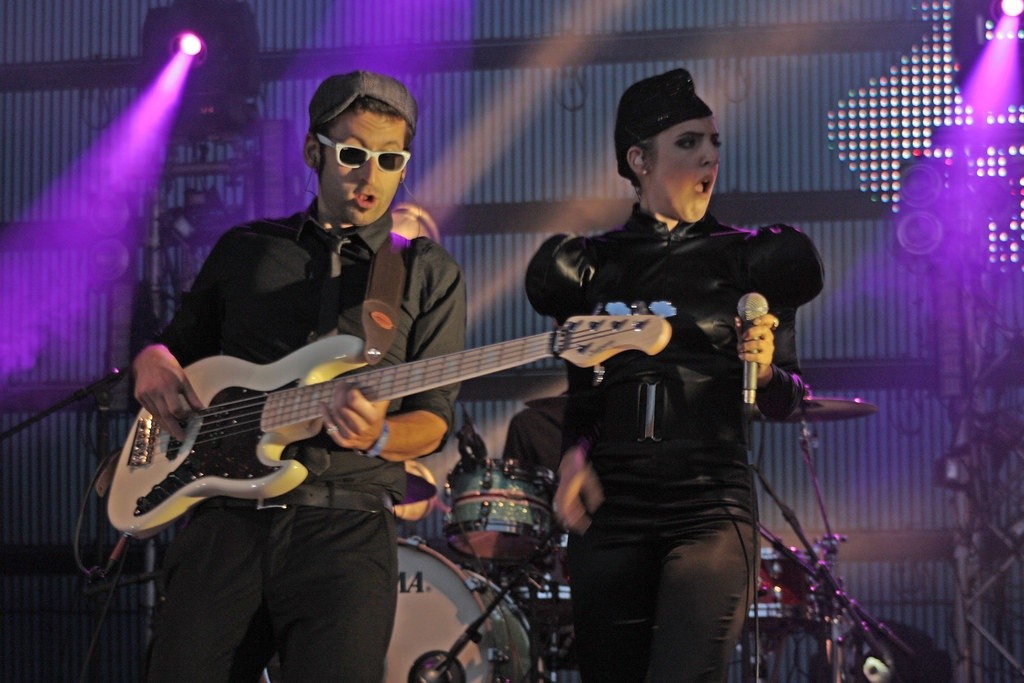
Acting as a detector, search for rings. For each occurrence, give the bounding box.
[326,426,338,434]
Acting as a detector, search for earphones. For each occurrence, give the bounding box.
[634,155,643,166]
[312,154,317,161]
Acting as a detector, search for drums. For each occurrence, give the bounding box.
[746,546,821,634]
[263,536,543,683]
[512,580,582,672]
[443,458,553,562]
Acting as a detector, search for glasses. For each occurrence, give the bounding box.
[317,132,412,175]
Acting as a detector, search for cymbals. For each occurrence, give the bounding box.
[751,396,878,423]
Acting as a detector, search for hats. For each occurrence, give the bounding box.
[309,70,418,138]
[614,67,714,156]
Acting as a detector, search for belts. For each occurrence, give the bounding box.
[199,483,396,514]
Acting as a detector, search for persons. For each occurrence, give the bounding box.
[502,68,825,683]
[133,68,468,683]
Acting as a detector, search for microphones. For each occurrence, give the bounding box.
[418,669,448,683]
[461,406,488,460]
[737,292,769,418]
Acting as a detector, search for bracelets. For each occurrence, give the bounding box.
[354,420,388,458]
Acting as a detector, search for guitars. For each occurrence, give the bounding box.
[105,294,678,539]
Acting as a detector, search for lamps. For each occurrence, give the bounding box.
[894,157,950,261]
[137,0,263,137]
